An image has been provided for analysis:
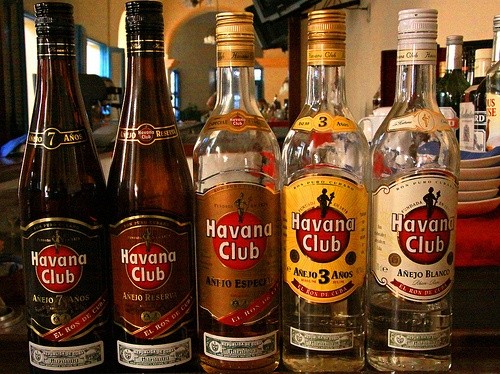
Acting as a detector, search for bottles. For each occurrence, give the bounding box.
[281,8,372,374]
[18,0,110,374]
[438,61,445,78]
[191,11,282,374]
[474,15,500,131]
[467,49,493,102]
[486,61,500,146]
[437,34,473,143]
[104,1,193,374]
[463,50,473,84]
[367,7,460,373]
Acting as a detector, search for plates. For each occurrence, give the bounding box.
[456,153,500,216]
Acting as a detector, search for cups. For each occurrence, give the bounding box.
[356,114,385,143]
